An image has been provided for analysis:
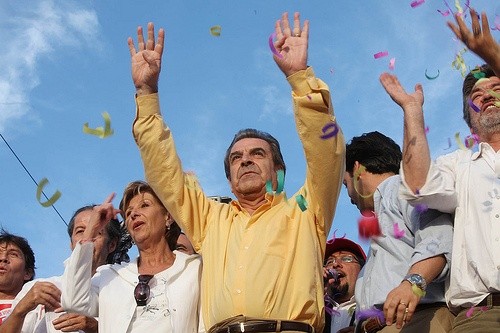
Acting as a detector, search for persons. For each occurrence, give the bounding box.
[128,11,345,333]
[322,8,500,333]
[0,180,232,333]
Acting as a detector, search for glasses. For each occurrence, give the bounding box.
[324,254,360,268]
[134,274,154,307]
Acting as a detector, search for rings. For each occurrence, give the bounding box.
[293,34,300,37]
[399,302,407,307]
[473,27,481,35]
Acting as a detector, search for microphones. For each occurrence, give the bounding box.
[324,268,338,284]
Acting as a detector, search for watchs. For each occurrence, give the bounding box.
[401,273,426,291]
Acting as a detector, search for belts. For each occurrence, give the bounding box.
[215,321,313,333]
[452,291,500,316]
[359,302,445,333]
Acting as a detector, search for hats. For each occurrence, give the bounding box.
[324,238,367,262]
